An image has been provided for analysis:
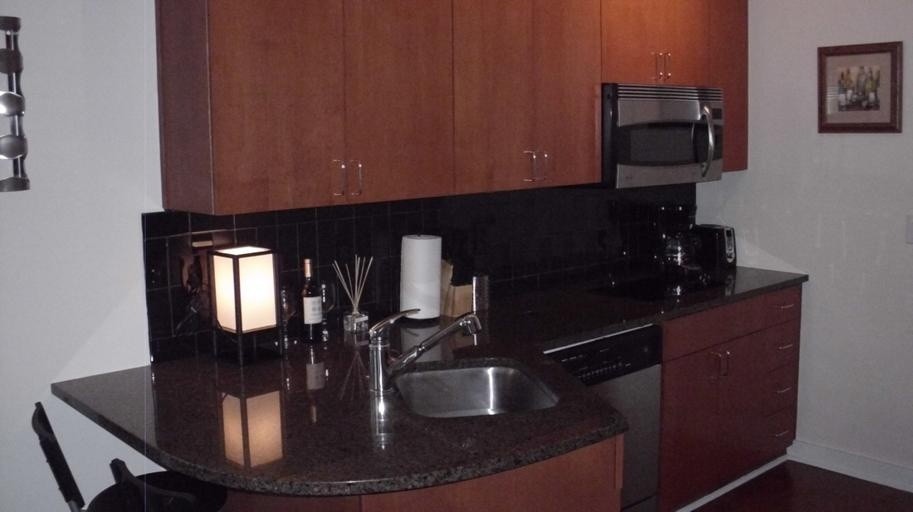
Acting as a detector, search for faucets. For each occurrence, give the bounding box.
[366,308,483,397]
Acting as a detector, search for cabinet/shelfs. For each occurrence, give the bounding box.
[602,1,748,172]
[156,0,451,215]
[659,284,802,510]
[452,1,602,196]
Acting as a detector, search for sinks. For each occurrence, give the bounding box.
[391,366,558,420]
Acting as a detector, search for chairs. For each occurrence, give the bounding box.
[110,458,197,512]
[32,402,228,512]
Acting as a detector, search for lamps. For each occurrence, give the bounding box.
[215,369,286,472]
[208,245,283,368]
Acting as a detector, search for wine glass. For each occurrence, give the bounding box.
[319,281,337,343]
[275,287,297,346]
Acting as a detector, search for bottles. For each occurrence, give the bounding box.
[303,345,327,426]
[836,66,879,107]
[298,259,324,344]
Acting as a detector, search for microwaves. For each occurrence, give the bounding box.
[606,83,724,190]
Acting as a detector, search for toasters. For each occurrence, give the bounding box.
[695,223,737,277]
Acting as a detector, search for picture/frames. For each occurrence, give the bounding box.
[817,41,902,133]
